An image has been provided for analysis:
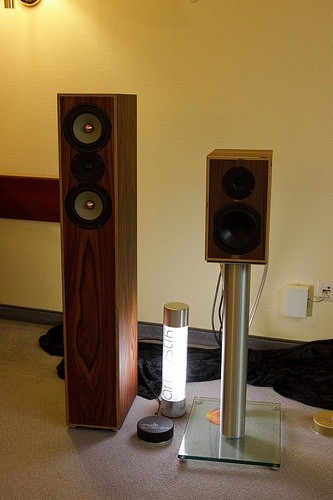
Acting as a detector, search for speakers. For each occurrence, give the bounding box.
[57,94,138,426]
[204,148,274,265]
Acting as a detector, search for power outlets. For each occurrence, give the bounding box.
[317,282,333,302]
